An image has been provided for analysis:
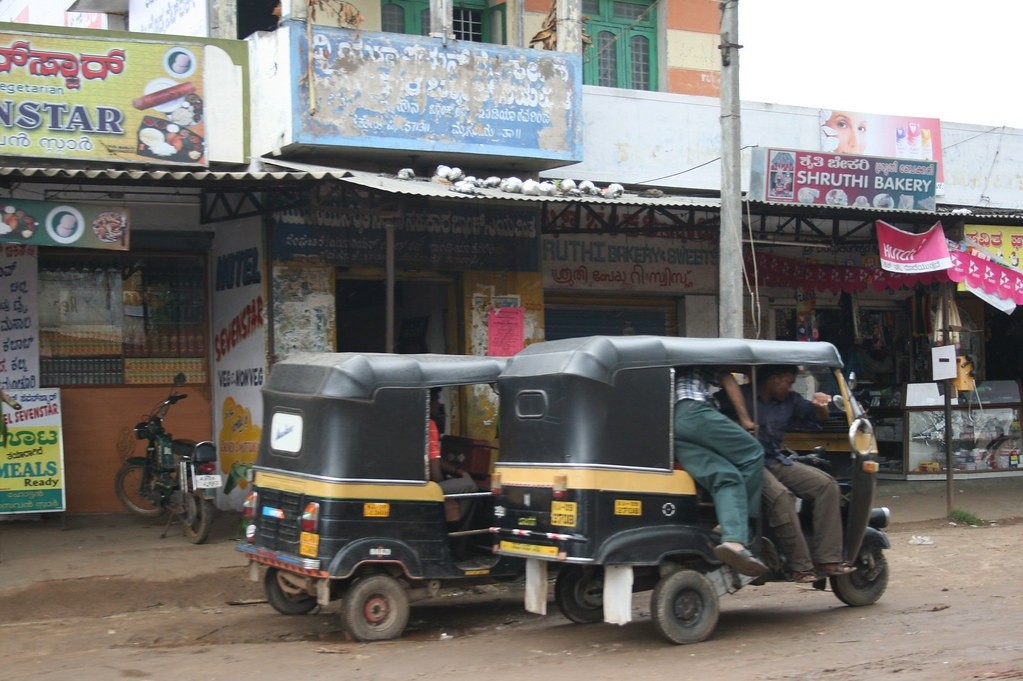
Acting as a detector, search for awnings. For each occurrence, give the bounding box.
[250,155,722,208]
[742,198,1023,219]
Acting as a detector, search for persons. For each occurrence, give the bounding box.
[428,418,478,560]
[428,387,446,433]
[674,365,770,577]
[822,110,868,155]
[830,328,866,395]
[716,364,858,582]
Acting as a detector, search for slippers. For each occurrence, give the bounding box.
[714,546,771,577]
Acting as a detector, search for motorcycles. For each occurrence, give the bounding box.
[491,335,890,647]
[115,373,224,546]
[237,351,516,644]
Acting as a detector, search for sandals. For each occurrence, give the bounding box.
[791,569,819,582]
[817,560,858,578]
